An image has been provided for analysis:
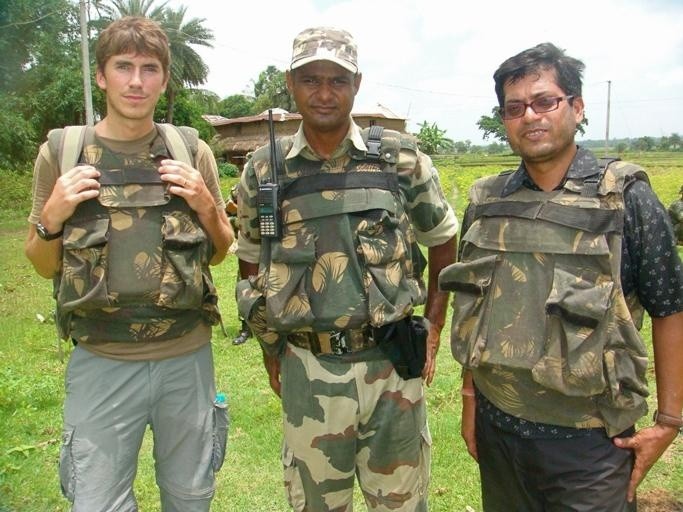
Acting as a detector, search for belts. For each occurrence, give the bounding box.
[289,329,374,357]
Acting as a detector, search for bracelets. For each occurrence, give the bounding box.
[652,409,683,432]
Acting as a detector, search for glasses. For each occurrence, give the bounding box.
[501,96,574,120]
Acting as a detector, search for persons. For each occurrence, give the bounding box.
[22,16,235,511]
[232,26,458,512]
[667,186,682,249]
[436,42,683,512]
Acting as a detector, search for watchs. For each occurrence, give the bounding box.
[35,221,64,241]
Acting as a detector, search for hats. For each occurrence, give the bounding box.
[289,27,358,75]
